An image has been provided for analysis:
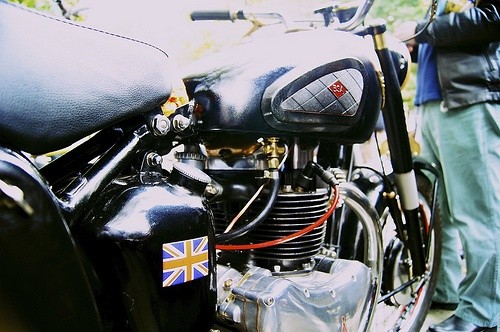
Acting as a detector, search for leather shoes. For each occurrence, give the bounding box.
[427,313,498,332]
[429,296,458,310]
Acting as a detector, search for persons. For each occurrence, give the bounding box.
[397,0,500,332]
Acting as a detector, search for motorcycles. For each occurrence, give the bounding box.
[0,0,445,330]
